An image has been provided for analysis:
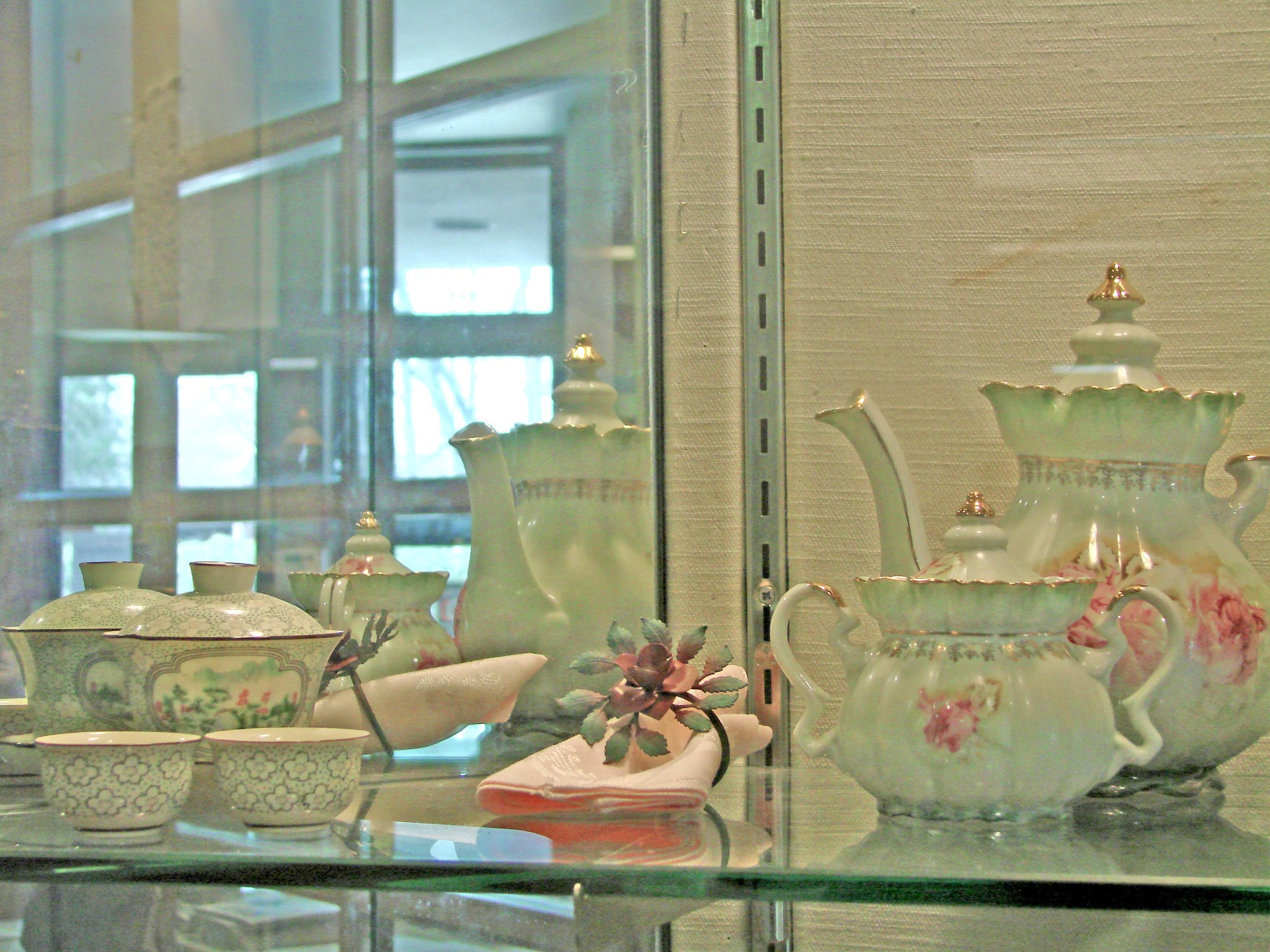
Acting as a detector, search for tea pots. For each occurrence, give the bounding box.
[813,262,1270,797]
[447,333,653,721]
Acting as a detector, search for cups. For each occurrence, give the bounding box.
[0,561,173,729]
[0,734,42,771]
[771,490,1185,816]
[203,727,371,836]
[34,730,202,844]
[286,511,460,692]
[102,560,345,758]
